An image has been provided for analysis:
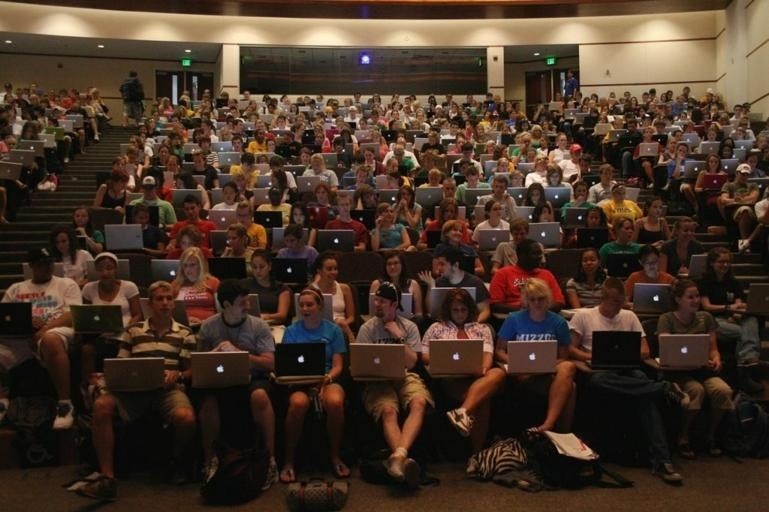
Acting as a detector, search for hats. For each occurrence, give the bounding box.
[570,144,583,153]
[736,163,752,175]
[28,248,59,262]
[376,282,404,312]
[141,176,157,185]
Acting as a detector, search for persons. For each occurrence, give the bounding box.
[0,70,769,503]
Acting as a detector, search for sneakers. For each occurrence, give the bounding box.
[205,456,221,482]
[466,454,478,478]
[446,409,472,437]
[381,453,406,480]
[738,240,752,255]
[81,477,118,501]
[53,400,75,428]
[653,462,681,481]
[404,458,420,490]
[263,468,278,489]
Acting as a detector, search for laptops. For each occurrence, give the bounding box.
[104,357,167,391]
[92,98,768,288]
[0,161,21,180]
[191,351,252,389]
[70,303,124,336]
[428,339,483,374]
[47,128,64,140]
[350,343,408,377]
[504,340,557,374]
[275,343,325,376]
[66,115,84,129]
[586,331,641,370]
[20,140,45,157]
[633,281,673,315]
[9,150,33,170]
[57,120,73,133]
[0,302,34,334]
[36,135,56,147]
[656,334,710,367]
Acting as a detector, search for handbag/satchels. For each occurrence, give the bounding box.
[287,478,348,512]
[360,442,427,485]
[625,177,644,188]
[478,433,600,493]
[202,450,268,505]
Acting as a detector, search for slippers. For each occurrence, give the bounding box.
[331,462,351,479]
[280,469,295,484]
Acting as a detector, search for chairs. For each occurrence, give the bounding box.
[2,84,768,455]
